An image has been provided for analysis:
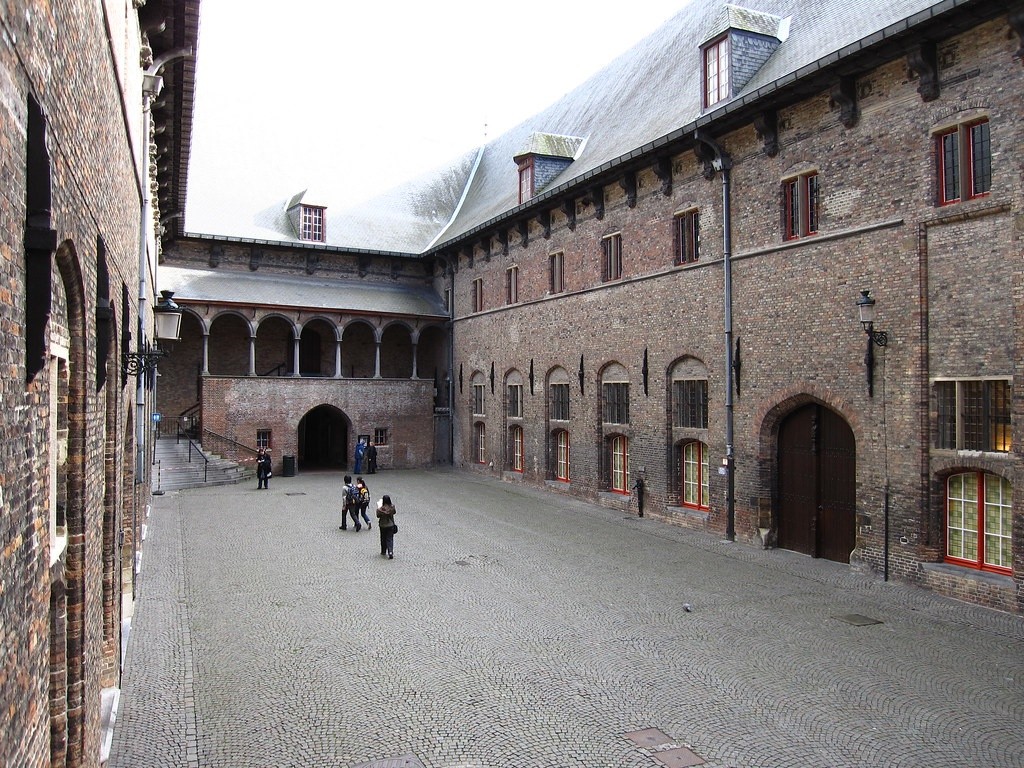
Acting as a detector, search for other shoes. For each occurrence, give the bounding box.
[389,552,393,559]
[356,523,361,532]
[339,526,346,530]
[382,553,386,555]
[368,521,371,529]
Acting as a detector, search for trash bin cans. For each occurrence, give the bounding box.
[282,455,295,477]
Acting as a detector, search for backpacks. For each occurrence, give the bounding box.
[357,486,370,508]
[345,484,359,506]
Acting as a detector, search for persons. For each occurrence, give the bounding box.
[376,495,396,559]
[356,476,371,529]
[366,442,377,474]
[256,449,272,489]
[339,474,361,532]
[354,440,365,474]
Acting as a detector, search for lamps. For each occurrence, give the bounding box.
[120,289,186,391]
[856,290,887,347]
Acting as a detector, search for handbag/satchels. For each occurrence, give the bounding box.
[393,524,398,533]
